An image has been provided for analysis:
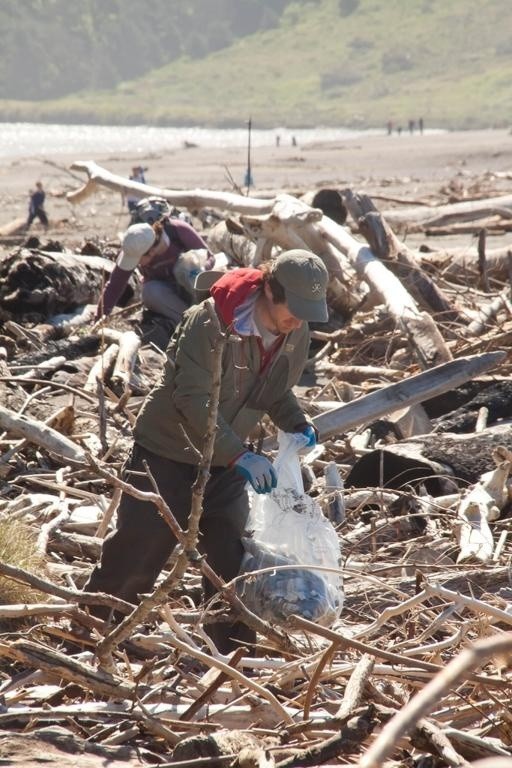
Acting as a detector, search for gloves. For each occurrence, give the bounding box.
[230,451,278,493]
[298,426,316,455]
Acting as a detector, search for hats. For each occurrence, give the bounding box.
[116,222,156,271]
[272,249,329,323]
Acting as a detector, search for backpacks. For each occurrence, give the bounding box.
[130,194,184,249]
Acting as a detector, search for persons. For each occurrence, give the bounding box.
[21,182,51,242]
[92,213,215,353]
[124,167,146,211]
[58,248,331,708]
